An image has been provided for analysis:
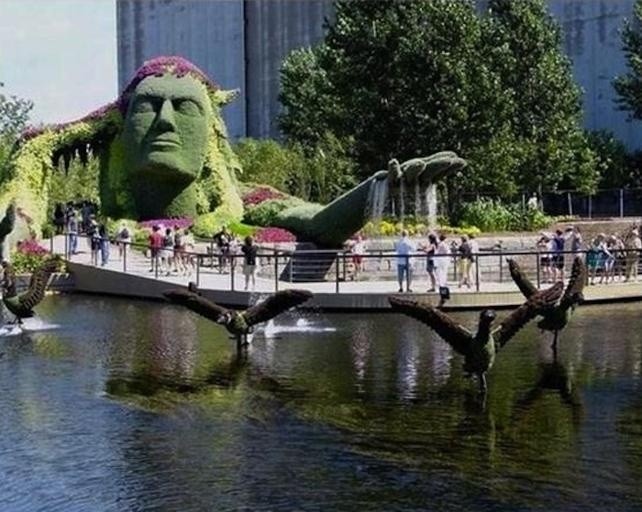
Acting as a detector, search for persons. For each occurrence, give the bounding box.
[54,200,132,267]
[391,229,479,293]
[212,226,233,275]
[343,233,368,284]
[240,235,260,291]
[147,225,196,277]
[1,55,467,246]
[535,221,642,284]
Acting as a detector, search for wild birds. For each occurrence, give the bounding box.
[388,282,564,394]
[0,257,66,324]
[163,288,312,351]
[506,256,587,345]
